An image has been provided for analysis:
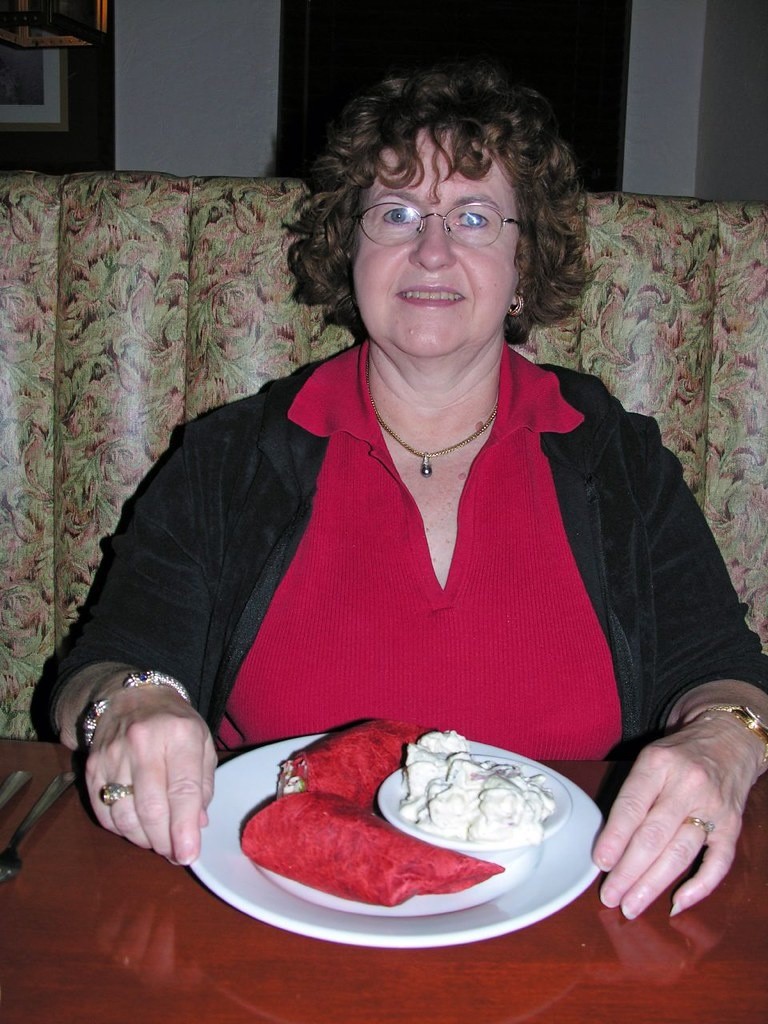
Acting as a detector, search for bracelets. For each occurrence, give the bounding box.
[81,671,192,747]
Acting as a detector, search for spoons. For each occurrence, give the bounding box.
[0,770,82,882]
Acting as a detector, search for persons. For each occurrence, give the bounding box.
[29,59,768,920]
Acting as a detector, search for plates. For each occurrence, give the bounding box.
[377,754,573,852]
[188,732,606,949]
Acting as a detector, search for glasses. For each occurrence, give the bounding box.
[350,202,527,249]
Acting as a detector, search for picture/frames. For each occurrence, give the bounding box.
[0,27,69,131]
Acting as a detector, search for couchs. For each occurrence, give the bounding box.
[0,166,768,742]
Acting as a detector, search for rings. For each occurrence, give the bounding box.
[100,782,133,805]
[685,816,715,843]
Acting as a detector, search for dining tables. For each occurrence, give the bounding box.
[0,739,768,1024]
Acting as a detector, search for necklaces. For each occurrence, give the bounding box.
[364,361,498,479]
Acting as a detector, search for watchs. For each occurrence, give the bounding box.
[706,703,768,768]
